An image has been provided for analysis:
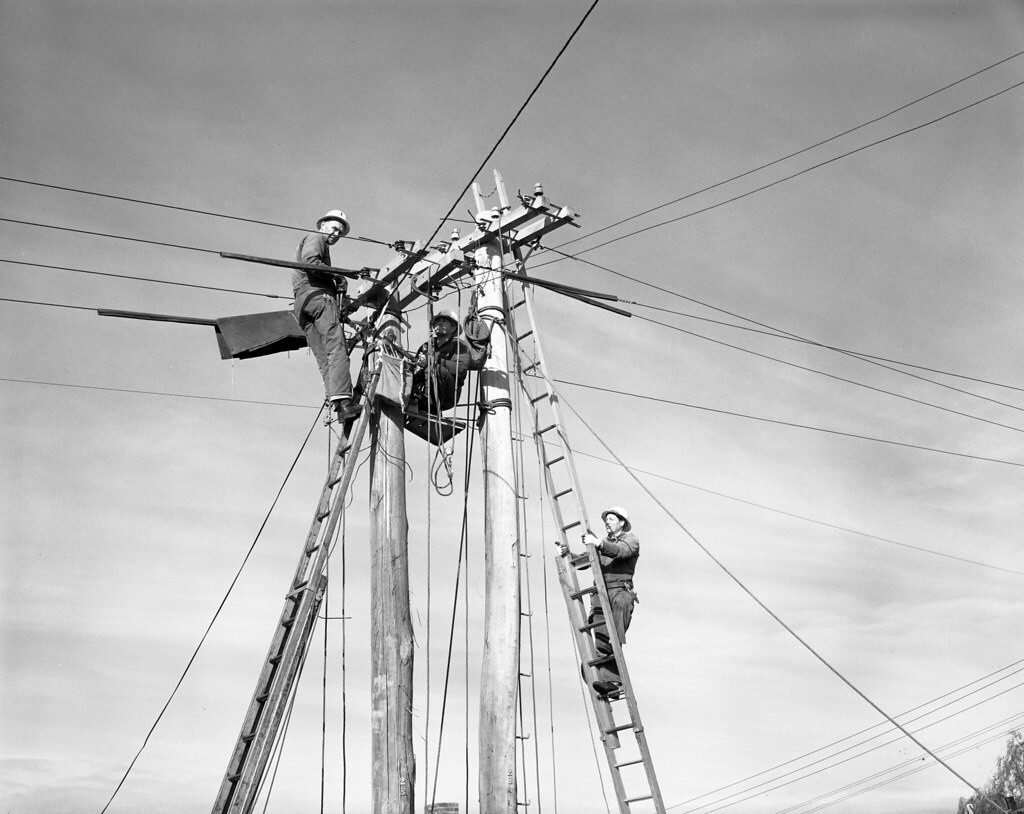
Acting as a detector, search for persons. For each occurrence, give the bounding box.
[406,310,472,414]
[293,210,363,423]
[555,506,640,700]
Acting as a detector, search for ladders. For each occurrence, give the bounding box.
[212,341,383,814]
[470,169,666,814]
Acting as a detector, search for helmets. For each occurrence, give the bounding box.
[602,506,631,533]
[316,210,350,237]
[431,309,463,337]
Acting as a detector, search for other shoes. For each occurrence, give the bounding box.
[593,681,620,700]
[597,643,614,656]
[338,399,362,423]
